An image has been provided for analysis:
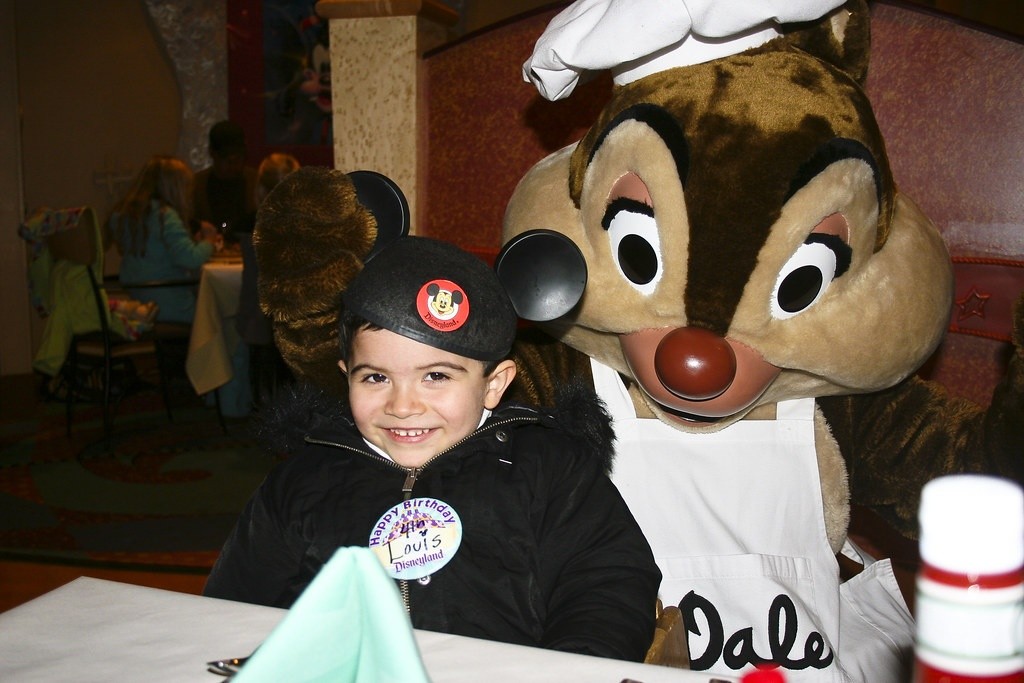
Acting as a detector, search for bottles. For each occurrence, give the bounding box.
[908,475,1024,683]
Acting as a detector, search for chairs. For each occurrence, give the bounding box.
[17,204,231,458]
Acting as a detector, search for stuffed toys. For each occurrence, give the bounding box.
[252,0,1024,683]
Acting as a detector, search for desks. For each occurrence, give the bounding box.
[0,575,744,683]
[185,244,290,438]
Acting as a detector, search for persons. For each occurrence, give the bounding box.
[205,234,663,661]
[189,121,260,241]
[254,154,300,206]
[100,159,223,324]
